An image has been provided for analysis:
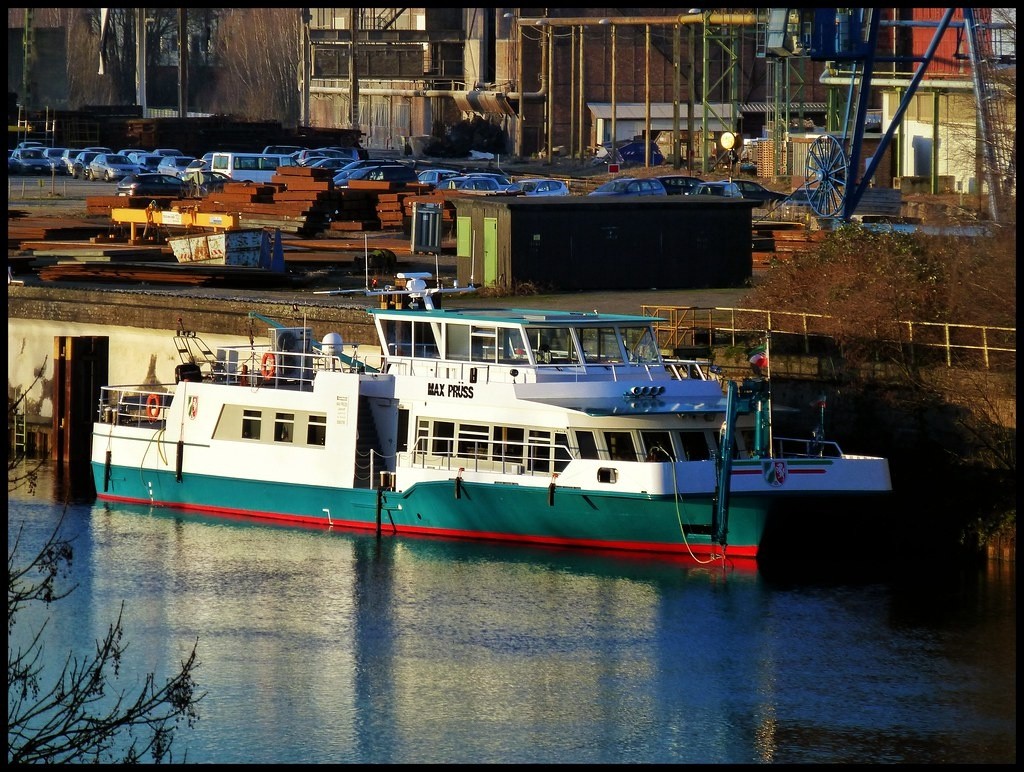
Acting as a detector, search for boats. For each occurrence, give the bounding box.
[92,232,893,557]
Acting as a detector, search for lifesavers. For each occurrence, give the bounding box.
[145,394,161,421]
[261,353,275,380]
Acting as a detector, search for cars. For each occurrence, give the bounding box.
[117,149,149,158]
[617,140,664,166]
[127,152,164,169]
[719,179,790,204]
[88,154,140,183]
[507,179,570,197]
[261,144,514,196]
[116,173,199,201]
[651,174,708,197]
[157,155,196,177]
[783,187,825,209]
[586,178,667,198]
[8,141,114,181]
[175,170,240,197]
[182,158,213,179]
[683,182,744,200]
[152,148,183,158]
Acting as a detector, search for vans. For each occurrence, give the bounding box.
[209,152,307,185]
